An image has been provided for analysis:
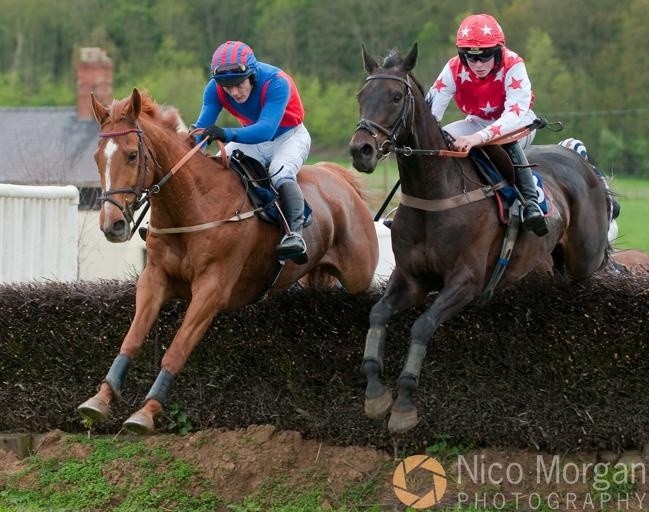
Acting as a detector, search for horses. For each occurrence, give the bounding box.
[349,43,629,433]
[78,87,379,436]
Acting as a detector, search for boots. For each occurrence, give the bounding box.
[139,227,147,241]
[275,182,308,265]
[504,139,549,237]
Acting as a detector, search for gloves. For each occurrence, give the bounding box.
[201,126,226,145]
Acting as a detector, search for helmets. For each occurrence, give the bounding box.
[456,14,505,70]
[210,40,256,86]
[558,137,588,161]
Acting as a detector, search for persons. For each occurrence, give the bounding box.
[188,40,312,265]
[424,12,550,237]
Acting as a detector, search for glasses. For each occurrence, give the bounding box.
[209,63,248,76]
[465,50,495,63]
